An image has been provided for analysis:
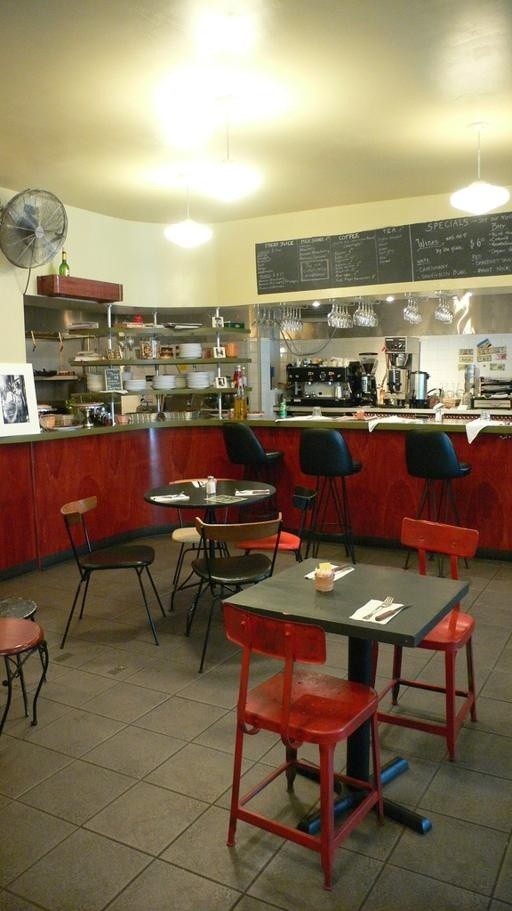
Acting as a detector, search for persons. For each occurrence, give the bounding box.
[13,379,28,421]
[3,392,23,422]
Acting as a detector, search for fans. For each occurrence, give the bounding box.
[1,190,70,270]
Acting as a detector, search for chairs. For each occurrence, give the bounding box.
[168,479,236,584]
[60,496,167,649]
[184,512,286,674]
[237,486,318,559]
[299,427,361,566]
[371,518,480,762]
[219,422,284,518]
[218,602,384,892]
[404,427,473,576]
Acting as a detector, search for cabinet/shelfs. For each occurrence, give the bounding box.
[21,296,257,419]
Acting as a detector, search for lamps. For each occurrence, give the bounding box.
[162,179,217,249]
[447,125,509,215]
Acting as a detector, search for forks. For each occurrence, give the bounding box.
[234,486,270,497]
[362,594,395,621]
[163,489,185,499]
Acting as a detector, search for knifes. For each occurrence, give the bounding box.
[375,602,414,621]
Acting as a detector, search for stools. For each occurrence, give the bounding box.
[0,595,38,619]
[0,619,51,731]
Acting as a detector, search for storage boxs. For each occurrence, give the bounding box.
[36,274,124,303]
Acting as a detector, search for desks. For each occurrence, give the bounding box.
[145,479,276,588]
[220,558,470,835]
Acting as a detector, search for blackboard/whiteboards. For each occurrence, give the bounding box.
[255,224,413,297]
[410,211,512,282]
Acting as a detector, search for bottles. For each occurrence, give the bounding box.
[435,408,443,423]
[84,410,94,428]
[234,390,241,419]
[241,367,247,388]
[92,407,112,425]
[205,476,216,494]
[59,247,70,277]
[240,389,247,420]
[233,365,243,389]
